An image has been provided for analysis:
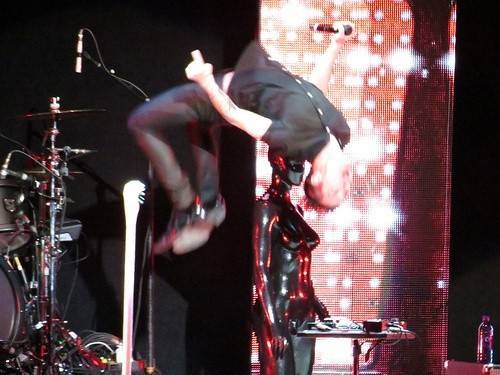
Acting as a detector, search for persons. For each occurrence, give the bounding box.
[254,145,331,375]
[127,20,352,255]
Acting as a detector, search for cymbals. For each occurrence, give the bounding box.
[21,107,105,123]
[25,169,83,176]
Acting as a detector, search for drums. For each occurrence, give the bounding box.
[0,170,34,256]
[0,256,28,351]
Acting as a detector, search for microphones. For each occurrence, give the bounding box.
[75,30,84,73]
[308,23,352,36]
[0,153,11,179]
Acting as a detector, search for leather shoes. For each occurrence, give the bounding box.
[172,191,227,256]
[150,195,202,256]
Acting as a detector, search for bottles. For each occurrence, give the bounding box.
[477,314,494,364]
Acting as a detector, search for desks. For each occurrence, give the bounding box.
[297,321,416,375]
